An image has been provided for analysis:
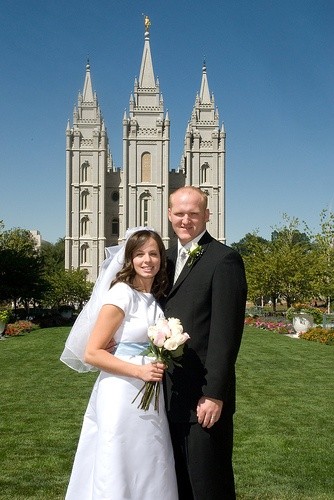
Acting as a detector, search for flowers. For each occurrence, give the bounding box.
[286,303,325,325]
[131,317,191,416]
[188,242,204,267]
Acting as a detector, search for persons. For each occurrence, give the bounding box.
[150,186,247,500]
[60,227,180,500]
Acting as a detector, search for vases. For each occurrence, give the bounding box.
[291,311,314,339]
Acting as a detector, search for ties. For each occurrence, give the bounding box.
[173,246,187,285]
